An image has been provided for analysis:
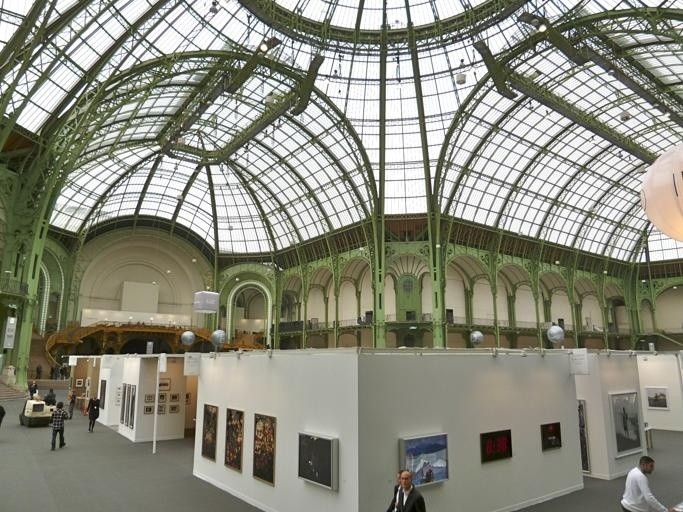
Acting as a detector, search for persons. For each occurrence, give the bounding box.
[386,470,425,512]
[620,408,630,440]
[33,364,68,379]
[84,395,100,433]
[0,380,75,451]
[619,456,675,512]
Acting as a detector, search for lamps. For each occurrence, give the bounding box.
[221,32,284,100]
[288,51,329,119]
[618,108,631,123]
[452,56,469,86]
[515,9,592,69]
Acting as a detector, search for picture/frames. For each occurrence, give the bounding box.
[74,377,192,430]
[253,412,277,488]
[201,403,219,463]
[607,390,643,462]
[574,397,591,472]
[398,432,449,489]
[224,407,244,475]
[644,386,672,411]
[295,428,339,492]
